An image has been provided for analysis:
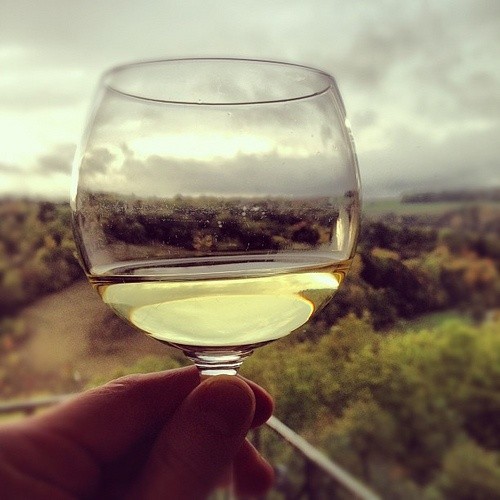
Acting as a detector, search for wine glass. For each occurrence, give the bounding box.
[70,56,362,500]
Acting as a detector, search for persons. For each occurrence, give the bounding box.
[0,362,277,499]
